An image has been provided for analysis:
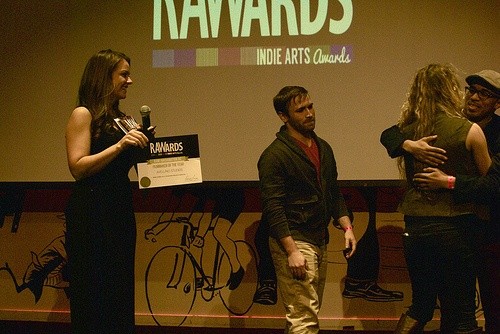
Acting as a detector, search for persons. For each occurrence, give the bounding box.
[391,64,491,334]
[381,70,500,334]
[66,50,149,334]
[257,86,357,334]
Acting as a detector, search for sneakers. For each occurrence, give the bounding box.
[253,279,277,304]
[342,281,403,302]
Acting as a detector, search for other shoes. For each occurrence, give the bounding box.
[195,278,205,288]
[229,267,245,290]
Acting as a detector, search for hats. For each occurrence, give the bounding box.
[465,70,500,88]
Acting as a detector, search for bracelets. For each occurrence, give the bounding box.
[344,226,352,231]
[448,176,454,188]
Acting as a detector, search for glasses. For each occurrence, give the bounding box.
[465,87,500,100]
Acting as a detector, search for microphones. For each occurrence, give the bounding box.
[140,105,151,138]
